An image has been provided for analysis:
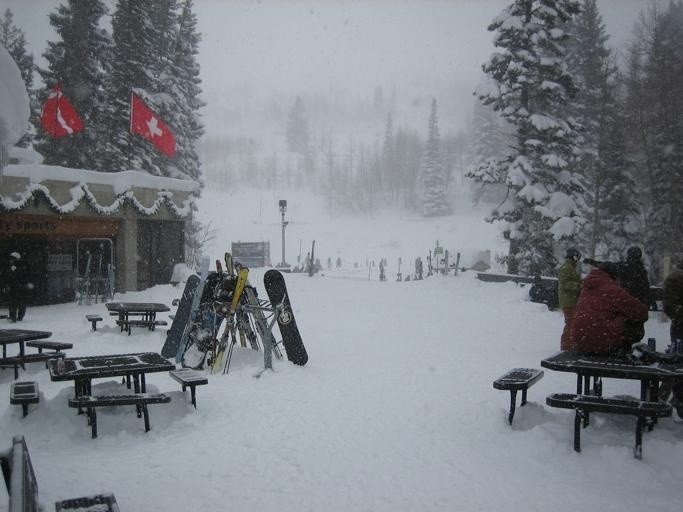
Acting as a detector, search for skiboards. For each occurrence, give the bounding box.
[79,252,102,304]
[211,252,282,378]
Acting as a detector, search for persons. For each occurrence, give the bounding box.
[661,252,683,419]
[558,248,582,351]
[572,262,649,355]
[4,252,28,322]
[583,247,657,311]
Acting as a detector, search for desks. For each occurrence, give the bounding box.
[541,350,683,433]
[105,302,170,329]
[48,353,175,414]
[0,329,52,369]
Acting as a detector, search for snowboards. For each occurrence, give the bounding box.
[161,271,224,369]
[445,250,461,275]
[263,269,307,366]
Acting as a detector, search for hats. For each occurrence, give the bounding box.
[10,252,21,262]
[567,246,644,275]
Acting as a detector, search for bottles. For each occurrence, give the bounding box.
[647,337,655,352]
[57,356,65,375]
[664,339,682,355]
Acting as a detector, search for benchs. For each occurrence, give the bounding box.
[169,368,208,410]
[86,311,176,336]
[11,381,39,417]
[68,393,171,438]
[493,366,544,425]
[54,493,119,512]
[594,376,651,397]
[0,339,73,380]
[545,392,673,459]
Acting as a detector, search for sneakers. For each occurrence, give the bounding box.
[6,317,23,324]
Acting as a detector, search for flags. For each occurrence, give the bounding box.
[41,83,83,137]
[131,91,175,158]
